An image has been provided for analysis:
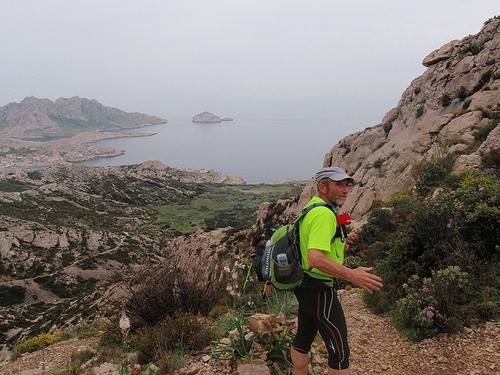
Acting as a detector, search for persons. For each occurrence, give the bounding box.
[252,224,276,301]
[290,166,384,375]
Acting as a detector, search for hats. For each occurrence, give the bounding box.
[315,167,355,186]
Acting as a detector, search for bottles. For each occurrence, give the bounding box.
[277,253,292,284]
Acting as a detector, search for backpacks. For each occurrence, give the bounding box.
[252,234,266,272]
[262,204,341,291]
